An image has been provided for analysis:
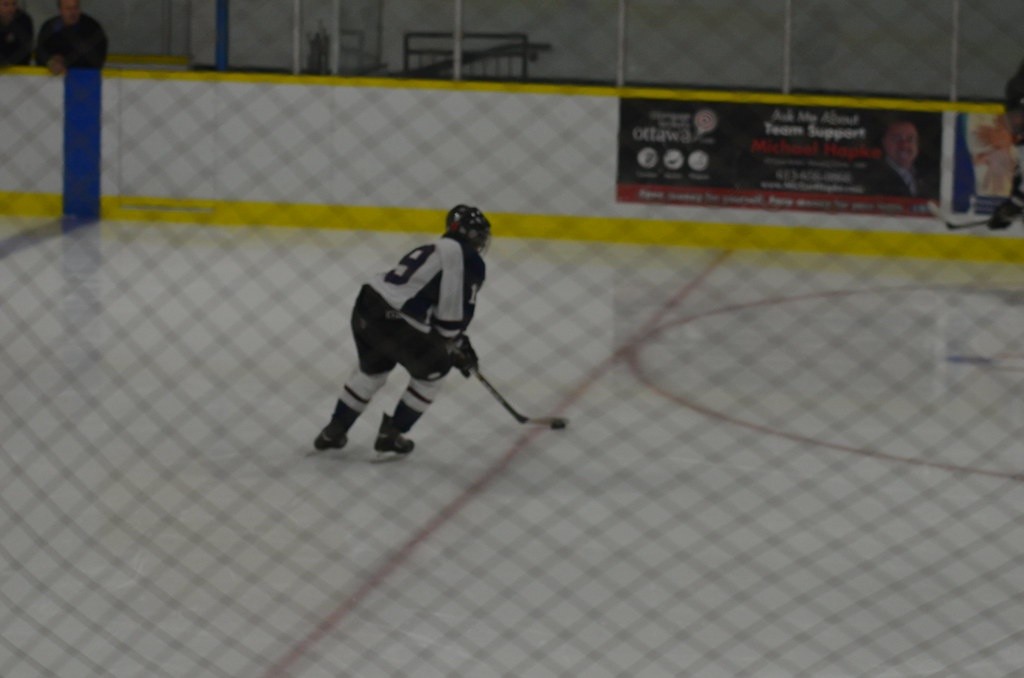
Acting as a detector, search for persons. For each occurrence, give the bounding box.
[35,0,108,75]
[865,120,937,200]
[987,60,1024,231]
[0,0,34,67]
[304,203,490,463]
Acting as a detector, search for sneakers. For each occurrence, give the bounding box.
[375,412,415,463]
[305,415,348,457]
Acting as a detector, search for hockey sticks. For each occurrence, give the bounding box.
[474,366,568,428]
[927,201,990,231]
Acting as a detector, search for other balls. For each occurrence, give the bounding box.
[550,420,566,430]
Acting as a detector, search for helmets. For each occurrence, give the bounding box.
[446,204,491,253]
[1000,76,1024,146]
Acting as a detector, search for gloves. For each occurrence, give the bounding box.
[987,199,1021,230]
[448,334,479,378]
